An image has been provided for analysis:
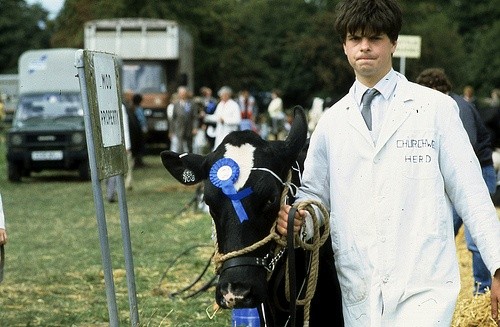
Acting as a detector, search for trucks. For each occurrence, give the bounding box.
[4,47,124,184]
[83,18,193,153]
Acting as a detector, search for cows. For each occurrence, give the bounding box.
[160,105,344,327]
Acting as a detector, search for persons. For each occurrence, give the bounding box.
[415,67,500,296]
[0,195,8,284]
[276,0,500,327]
[106,86,331,204]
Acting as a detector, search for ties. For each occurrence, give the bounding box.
[361,88,381,131]
